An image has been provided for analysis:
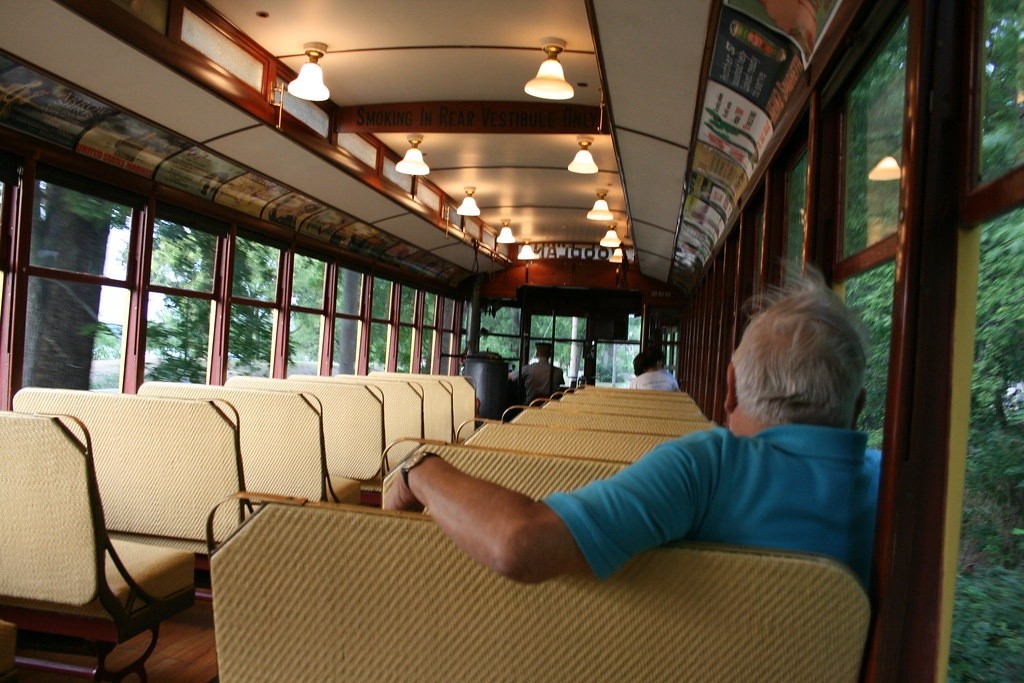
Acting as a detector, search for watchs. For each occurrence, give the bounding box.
[401,451,439,489]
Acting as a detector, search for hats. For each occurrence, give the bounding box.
[534,343,552,352]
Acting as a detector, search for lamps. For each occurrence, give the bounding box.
[496,218,515,244]
[600,222,621,247]
[568,135,598,175]
[587,188,613,220]
[395,133,430,175]
[517,238,539,260]
[457,185,480,216]
[524,35,576,100]
[609,243,626,263]
[287,42,330,101]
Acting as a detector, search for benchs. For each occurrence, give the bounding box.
[0,371,870,683]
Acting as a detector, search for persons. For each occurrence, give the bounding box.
[628,345,679,391]
[508,342,565,407]
[381,266,882,586]
